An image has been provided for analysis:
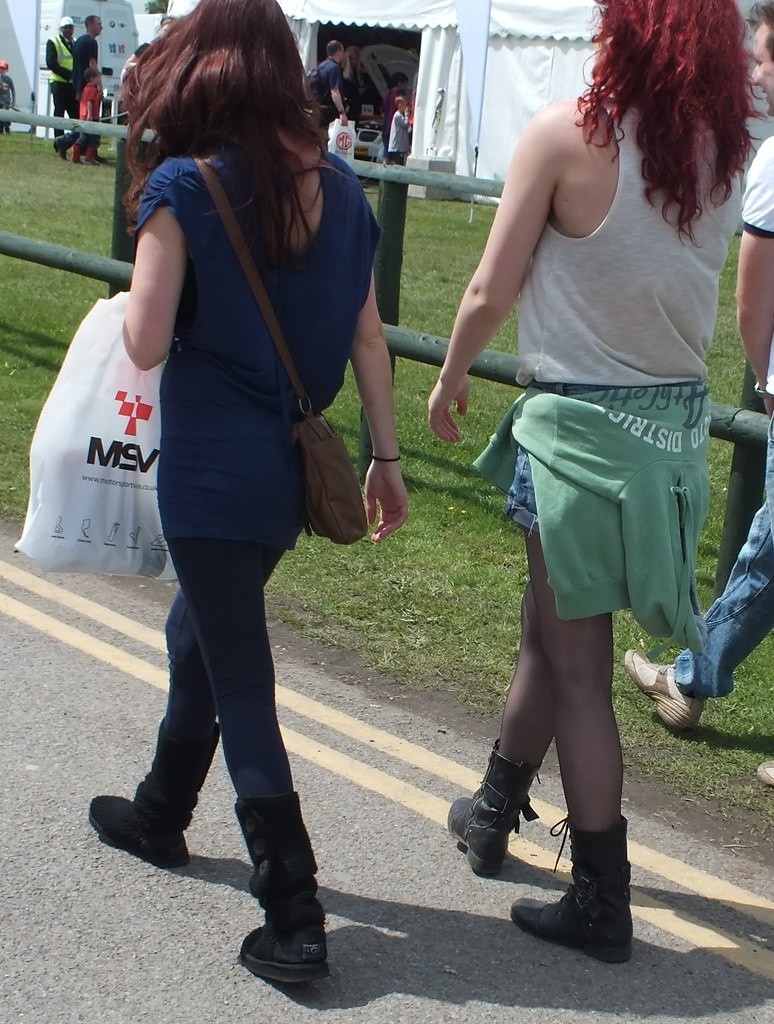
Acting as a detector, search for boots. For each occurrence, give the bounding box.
[88,716,220,869]
[53,139,103,165]
[448,740,543,878]
[509,814,632,964]
[234,792,329,984]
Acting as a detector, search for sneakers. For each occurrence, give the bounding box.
[757,761,774,787]
[624,650,704,733]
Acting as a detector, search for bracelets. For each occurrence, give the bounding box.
[371,454,401,462]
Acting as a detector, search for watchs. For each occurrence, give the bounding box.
[754,381,774,398]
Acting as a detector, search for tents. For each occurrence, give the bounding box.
[157,0,469,192]
[469,0,771,208]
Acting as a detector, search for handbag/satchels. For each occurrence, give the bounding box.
[297,408,368,544]
[16,291,177,581]
[327,118,355,161]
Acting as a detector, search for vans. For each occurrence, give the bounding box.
[38,0,138,105]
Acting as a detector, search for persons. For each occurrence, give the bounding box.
[620,0,774,786]
[88,0,406,986]
[72,68,103,163]
[0,59,16,134]
[46,16,77,152]
[55,15,103,159]
[307,39,419,166]
[427,0,762,959]
[125,17,175,73]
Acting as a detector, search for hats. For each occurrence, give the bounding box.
[0,59,9,69]
[59,16,74,28]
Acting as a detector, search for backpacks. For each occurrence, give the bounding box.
[307,69,321,105]
